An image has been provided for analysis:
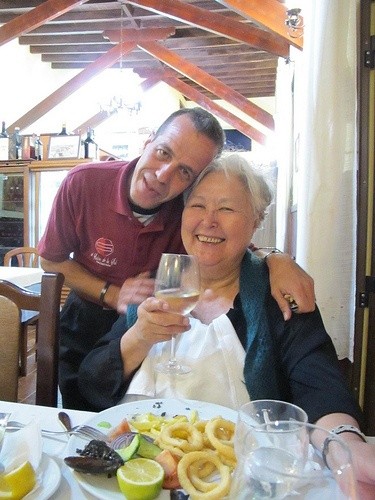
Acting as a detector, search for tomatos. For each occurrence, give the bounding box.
[112,417,131,438]
[155,449,180,489]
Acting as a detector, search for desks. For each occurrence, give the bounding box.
[0,266,46,377]
[0,401,375,500]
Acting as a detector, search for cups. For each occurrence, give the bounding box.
[233,399,309,465]
[228,419,357,500]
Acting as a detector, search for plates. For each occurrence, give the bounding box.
[0,451,62,500]
[66,397,271,500]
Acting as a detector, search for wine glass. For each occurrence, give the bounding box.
[0,403,19,475]
[154,252,202,376]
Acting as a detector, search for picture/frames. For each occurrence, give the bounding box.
[46,134,80,158]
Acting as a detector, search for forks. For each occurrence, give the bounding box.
[3,420,107,441]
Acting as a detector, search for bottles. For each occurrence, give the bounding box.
[13,127,23,159]
[31,133,43,161]
[84,126,97,159]
[58,123,70,136]
[7,186,22,201]
[0,121,10,138]
[76,129,85,159]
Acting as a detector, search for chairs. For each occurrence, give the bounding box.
[0,272,65,408]
[4,247,40,268]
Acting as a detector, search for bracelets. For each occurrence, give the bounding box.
[100,284,110,302]
[264,250,275,260]
[322,425,367,469]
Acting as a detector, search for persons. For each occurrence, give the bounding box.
[32,105,317,411]
[76,154,375,500]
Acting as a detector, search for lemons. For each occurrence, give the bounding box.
[0,461,36,500]
[116,457,165,500]
